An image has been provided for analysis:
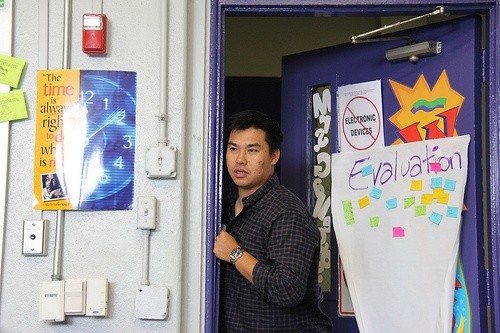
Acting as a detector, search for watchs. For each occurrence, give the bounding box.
[229,246,245,264]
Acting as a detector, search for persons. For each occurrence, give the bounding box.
[212,110,335,333]
[42,174,66,199]
